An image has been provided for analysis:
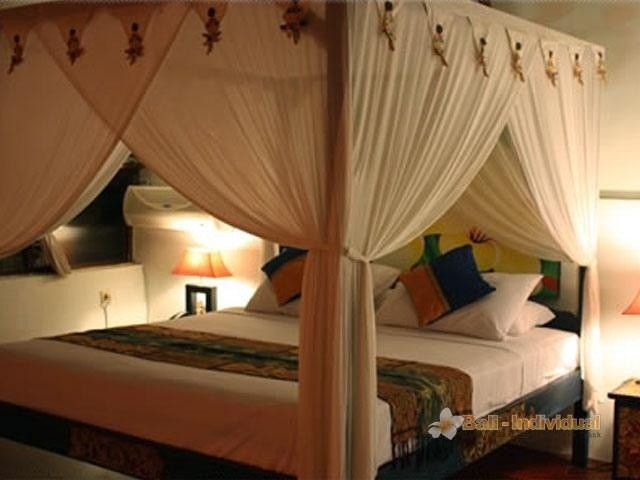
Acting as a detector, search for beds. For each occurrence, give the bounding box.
[0,0,606,480]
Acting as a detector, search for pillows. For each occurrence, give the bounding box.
[398,244,495,331]
[376,272,544,340]
[244,261,401,319]
[261,248,308,306]
[508,299,556,335]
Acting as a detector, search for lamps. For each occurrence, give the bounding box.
[623,292,640,315]
[168,248,234,315]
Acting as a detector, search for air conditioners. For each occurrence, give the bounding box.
[123,186,233,233]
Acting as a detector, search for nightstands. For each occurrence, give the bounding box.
[606,378,640,480]
